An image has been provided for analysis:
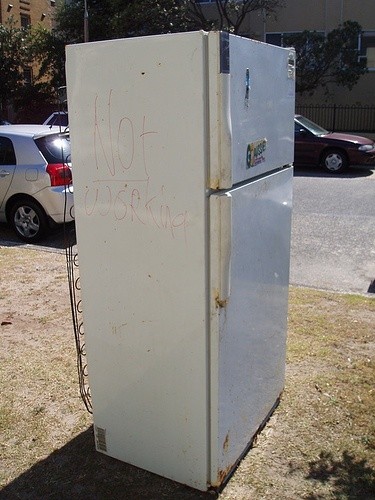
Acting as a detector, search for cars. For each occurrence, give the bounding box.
[293,113,374,174]
[1,120,76,242]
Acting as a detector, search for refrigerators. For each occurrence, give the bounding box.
[65,30,297,495]
